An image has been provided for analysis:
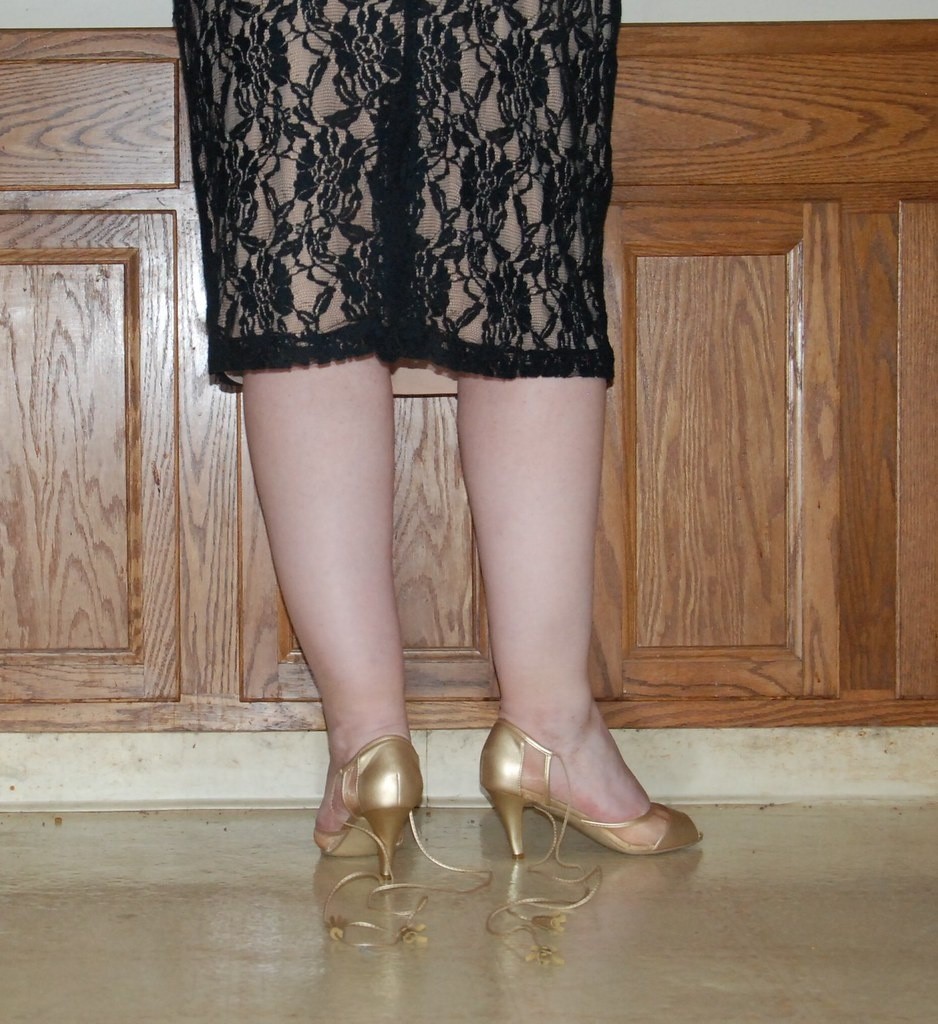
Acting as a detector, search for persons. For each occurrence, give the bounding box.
[163,0,708,881]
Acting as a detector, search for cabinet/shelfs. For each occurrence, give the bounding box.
[0,18,938,734]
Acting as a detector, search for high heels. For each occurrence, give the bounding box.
[480,718,704,861]
[313,734,424,880]
